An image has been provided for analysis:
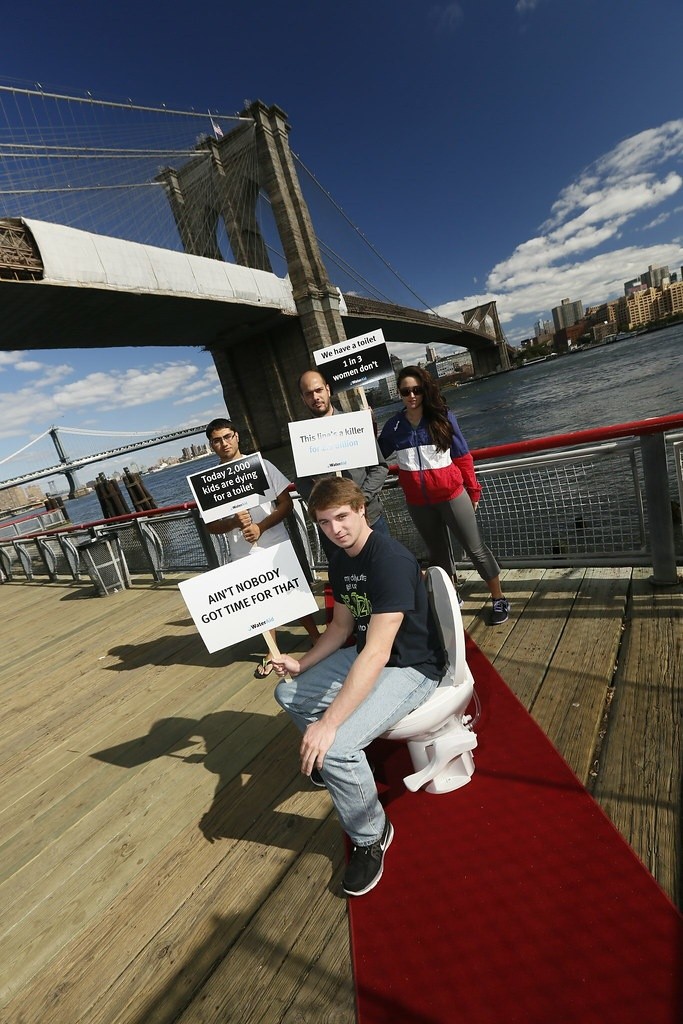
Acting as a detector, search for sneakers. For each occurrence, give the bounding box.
[311,760,375,787]
[341,812,393,896]
[491,596,511,624]
[456,594,464,607]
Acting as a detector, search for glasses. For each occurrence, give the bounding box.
[209,432,236,447]
[398,386,423,397]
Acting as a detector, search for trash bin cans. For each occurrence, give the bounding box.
[76,532,133,598]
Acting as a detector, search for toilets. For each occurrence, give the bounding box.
[375,566,483,795]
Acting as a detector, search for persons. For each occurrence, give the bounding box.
[378,363,510,624]
[293,370,390,561]
[272,477,447,894]
[203,418,321,679]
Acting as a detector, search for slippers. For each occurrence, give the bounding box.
[254,658,273,679]
